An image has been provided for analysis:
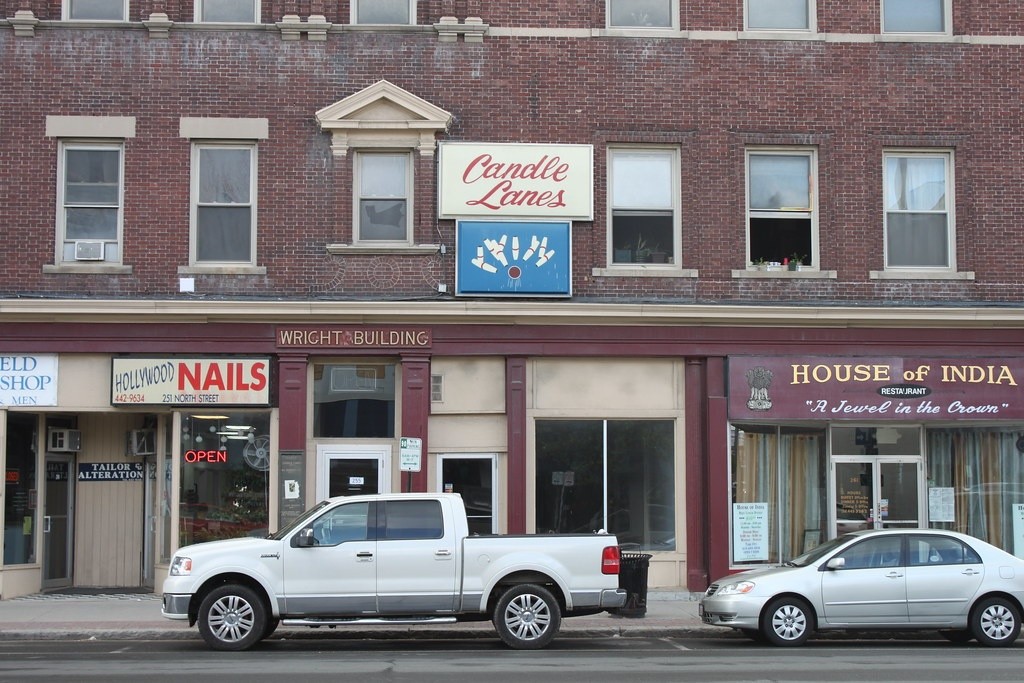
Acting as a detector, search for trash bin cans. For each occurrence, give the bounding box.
[608,553,652,618]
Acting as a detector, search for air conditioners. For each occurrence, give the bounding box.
[128,427,158,457]
[47,426,83,453]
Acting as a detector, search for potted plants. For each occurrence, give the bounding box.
[788,251,807,271]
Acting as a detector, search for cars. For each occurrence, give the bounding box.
[639,534,675,551]
[179,503,269,542]
[699,528,1024,647]
[836,503,874,538]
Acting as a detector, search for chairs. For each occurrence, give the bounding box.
[880,552,898,566]
[942,548,979,562]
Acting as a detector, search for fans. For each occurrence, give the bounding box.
[242,433,271,471]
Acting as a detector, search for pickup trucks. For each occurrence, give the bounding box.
[161,492,627,650]
